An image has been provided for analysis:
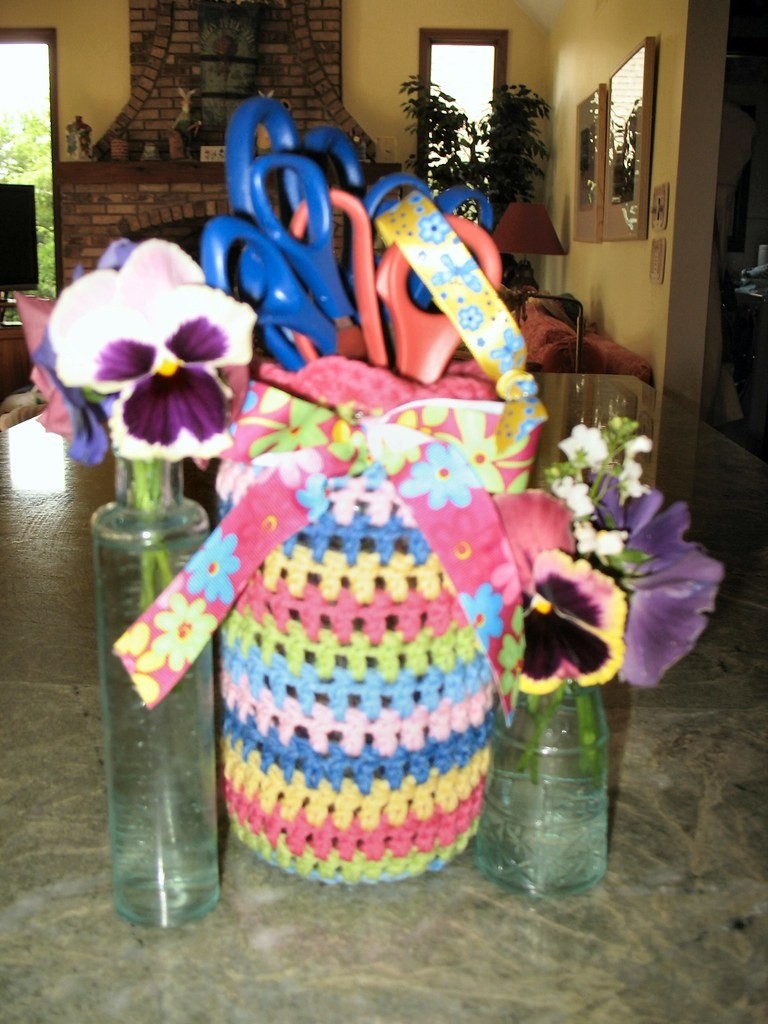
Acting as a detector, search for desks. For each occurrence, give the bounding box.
[0,374,768,1024]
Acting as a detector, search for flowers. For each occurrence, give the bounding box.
[15,239,257,679]
[516,418,727,786]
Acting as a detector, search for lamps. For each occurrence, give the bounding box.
[489,200,567,287]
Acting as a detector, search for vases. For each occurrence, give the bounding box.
[89,450,223,929]
[477,683,610,899]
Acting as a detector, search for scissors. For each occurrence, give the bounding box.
[200,95,502,384]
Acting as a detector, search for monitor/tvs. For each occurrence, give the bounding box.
[0,184,40,291]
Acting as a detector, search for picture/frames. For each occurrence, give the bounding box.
[571,82,608,243]
[603,35,656,240]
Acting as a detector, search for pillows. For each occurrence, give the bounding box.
[520,301,578,374]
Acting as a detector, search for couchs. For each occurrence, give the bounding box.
[508,291,651,383]
